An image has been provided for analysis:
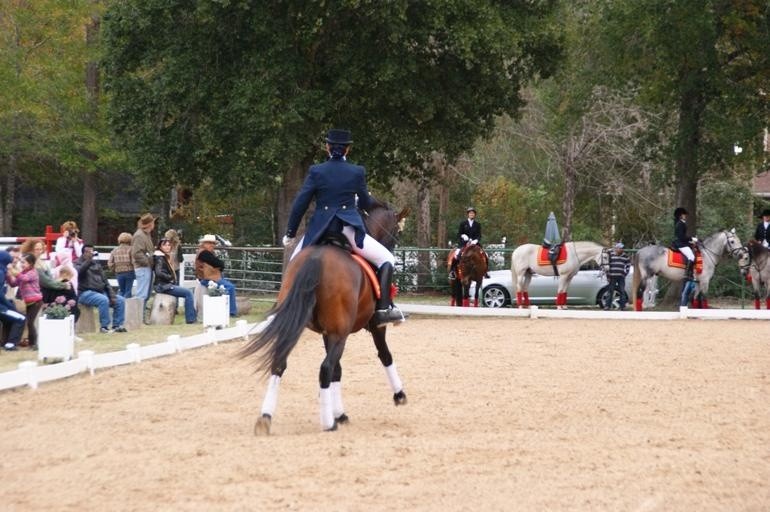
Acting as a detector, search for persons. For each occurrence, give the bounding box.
[602,242,630,311]
[280,130,410,326]
[0,211,240,350]
[671,206,696,277]
[680,282,695,312]
[448,206,490,279]
[747,210,770,250]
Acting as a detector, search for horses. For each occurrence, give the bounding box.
[232,197,412,436]
[740,239,770,309]
[447,245,488,307]
[510,240,614,310]
[632,227,749,311]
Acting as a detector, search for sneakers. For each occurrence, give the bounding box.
[186,319,193,324]
[100,327,111,334]
[112,326,127,332]
[604,304,626,310]
[0,337,37,351]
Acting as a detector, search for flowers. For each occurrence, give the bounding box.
[42,295,76,319]
[207,280,228,297]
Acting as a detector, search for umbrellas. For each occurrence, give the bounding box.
[541,211,561,244]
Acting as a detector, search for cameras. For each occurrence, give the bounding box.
[92,251,99,256]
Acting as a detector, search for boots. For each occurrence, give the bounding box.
[683,260,695,282]
[448,257,457,279]
[375,261,410,328]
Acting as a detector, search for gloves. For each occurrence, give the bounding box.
[691,237,698,243]
[282,234,297,248]
[461,233,470,242]
[471,238,479,245]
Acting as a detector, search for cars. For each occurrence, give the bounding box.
[87,243,118,261]
[466,245,643,313]
[390,242,505,283]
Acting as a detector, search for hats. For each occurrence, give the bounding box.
[325,129,354,144]
[760,208,769,216]
[198,234,220,244]
[467,207,477,213]
[137,212,159,227]
[674,207,689,217]
[615,243,624,249]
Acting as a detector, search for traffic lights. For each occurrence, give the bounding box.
[170,185,195,218]
